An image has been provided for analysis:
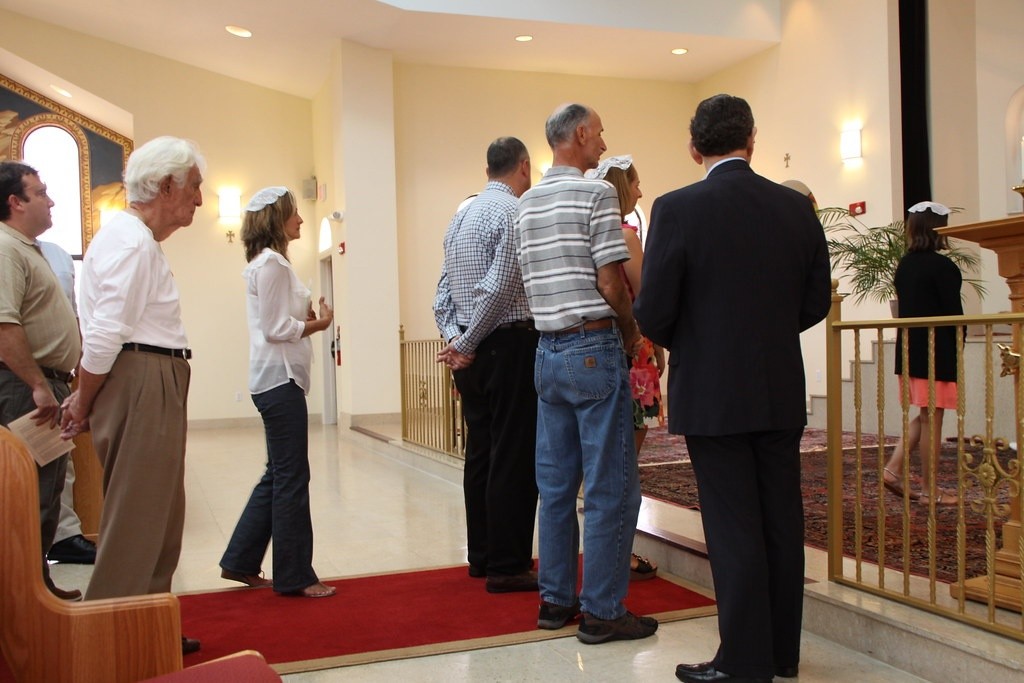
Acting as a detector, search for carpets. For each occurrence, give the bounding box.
[638,424,1018,583]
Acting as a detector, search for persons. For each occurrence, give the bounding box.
[882,202,967,508]
[431,134,537,593]
[55,134,201,658]
[511,102,666,647]
[218,186,340,598]
[631,92,830,683]
[0,162,98,601]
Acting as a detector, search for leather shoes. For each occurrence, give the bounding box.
[181,634,201,655]
[774,663,799,677]
[468,558,539,593]
[46,535,97,564]
[675,646,774,683]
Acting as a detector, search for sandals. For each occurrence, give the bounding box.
[630,552,657,581]
[221,567,274,587]
[291,580,337,598]
[882,468,921,501]
[917,486,959,507]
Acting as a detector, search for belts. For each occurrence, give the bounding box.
[0,360,74,383]
[123,342,192,359]
[558,318,612,334]
[454,321,534,332]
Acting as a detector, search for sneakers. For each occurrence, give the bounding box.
[575,606,658,644]
[537,597,580,629]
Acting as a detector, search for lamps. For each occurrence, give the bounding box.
[219,188,241,217]
[839,131,862,161]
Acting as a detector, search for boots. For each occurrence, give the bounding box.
[43,563,83,602]
